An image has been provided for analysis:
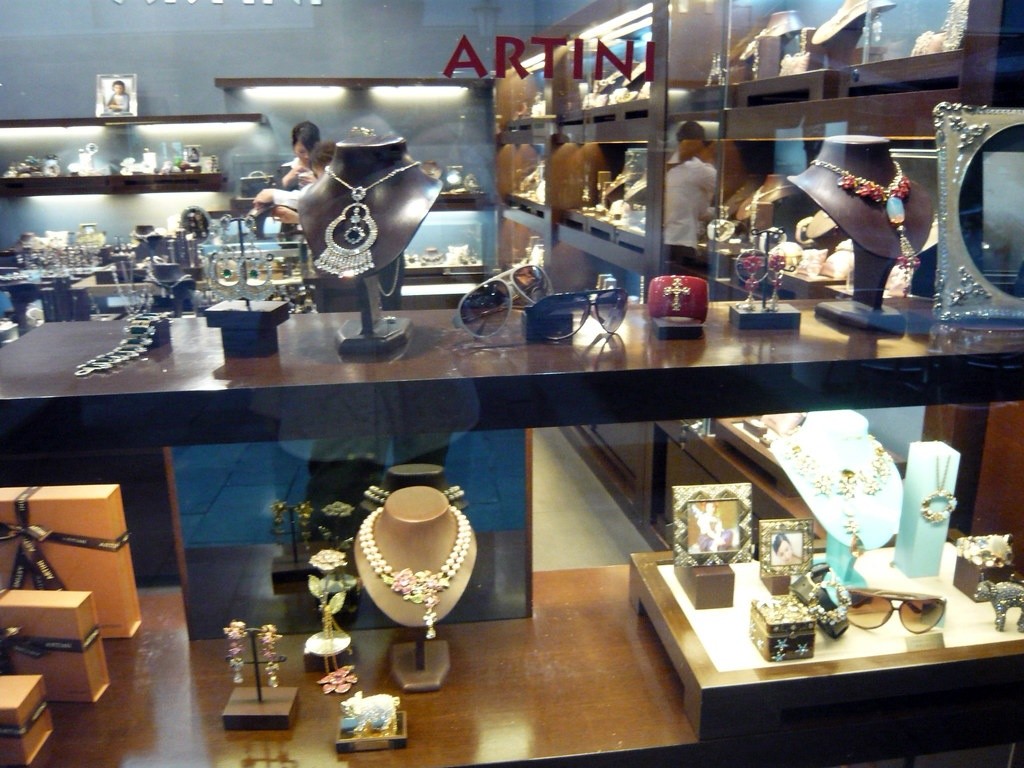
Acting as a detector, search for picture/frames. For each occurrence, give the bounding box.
[672,482,753,569]
[94,73,139,117]
[758,517,814,579]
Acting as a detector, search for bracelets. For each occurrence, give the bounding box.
[319,605,358,694]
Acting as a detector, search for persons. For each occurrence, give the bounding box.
[697,503,733,553]
[534,133,569,204]
[773,534,803,565]
[253,120,405,312]
[106,80,129,112]
[189,148,199,162]
[663,122,718,274]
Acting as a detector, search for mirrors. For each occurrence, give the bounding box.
[932,99,1024,324]
[162,427,534,643]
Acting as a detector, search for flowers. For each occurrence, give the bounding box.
[308,542,357,640]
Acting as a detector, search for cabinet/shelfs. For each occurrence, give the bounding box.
[216,78,496,286]
[494,0,1024,551]
[0,113,268,284]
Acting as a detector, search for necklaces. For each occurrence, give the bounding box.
[113,268,151,316]
[787,427,893,559]
[809,158,921,299]
[922,455,957,525]
[359,504,471,639]
[18,238,34,250]
[835,0,872,25]
[746,23,779,51]
[663,275,692,311]
[743,184,796,211]
[608,173,646,190]
[149,272,191,298]
[314,161,421,278]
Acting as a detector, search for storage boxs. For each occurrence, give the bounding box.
[0,674,54,765]
[0,484,142,639]
[953,553,1013,603]
[749,593,818,662]
[673,565,736,610]
[0,588,111,703]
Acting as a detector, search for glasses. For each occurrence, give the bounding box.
[528,287,629,341]
[455,262,555,341]
[842,589,948,635]
